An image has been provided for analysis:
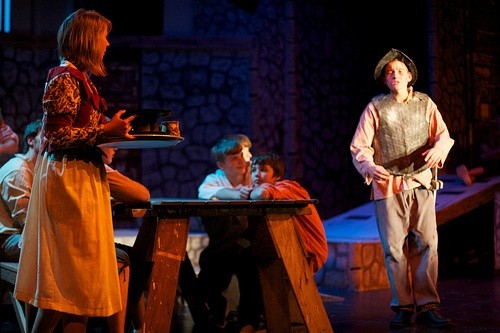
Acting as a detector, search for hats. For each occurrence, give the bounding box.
[374,49,418,87]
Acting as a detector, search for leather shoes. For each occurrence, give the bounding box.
[416,307,453,326]
[390,306,412,326]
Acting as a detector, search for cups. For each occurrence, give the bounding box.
[162,121,180,136]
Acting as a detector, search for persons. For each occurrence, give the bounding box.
[0,119,142,333]
[198,133,267,333]
[350,48,454,333]
[0,112,18,169]
[13,5,136,333]
[92,115,150,204]
[240,151,328,281]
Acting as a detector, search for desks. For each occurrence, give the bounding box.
[114,199,334,333]
